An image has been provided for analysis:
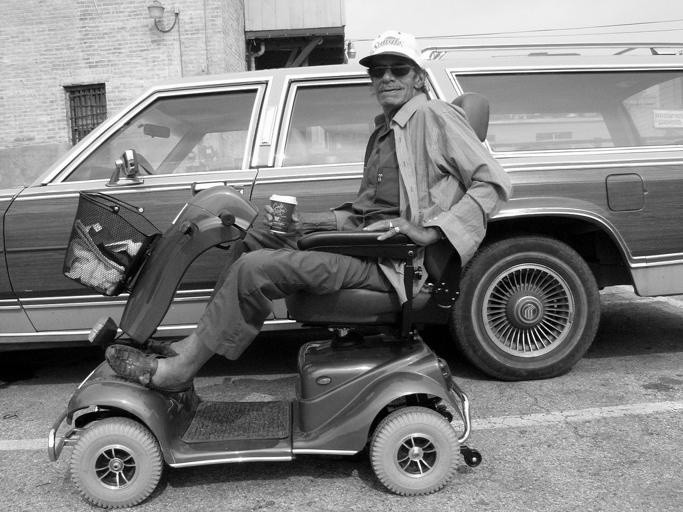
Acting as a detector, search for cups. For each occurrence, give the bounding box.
[269,194,298,235]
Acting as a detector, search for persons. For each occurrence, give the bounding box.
[105,29,512,394]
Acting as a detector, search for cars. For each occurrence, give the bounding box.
[2,59,682,381]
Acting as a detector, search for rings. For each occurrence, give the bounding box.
[395,227,400,233]
[389,220,394,230]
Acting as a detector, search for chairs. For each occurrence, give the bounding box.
[283,91,490,330]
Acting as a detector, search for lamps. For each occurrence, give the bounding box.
[147,0,180,33]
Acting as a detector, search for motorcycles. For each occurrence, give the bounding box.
[47,190,481,509]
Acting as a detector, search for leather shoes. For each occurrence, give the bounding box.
[141,339,179,356]
[106,345,192,394]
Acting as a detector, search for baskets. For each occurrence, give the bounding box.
[63,190,162,296]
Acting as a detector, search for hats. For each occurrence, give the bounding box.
[359,31,426,70]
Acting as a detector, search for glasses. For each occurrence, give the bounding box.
[367,65,414,77]
